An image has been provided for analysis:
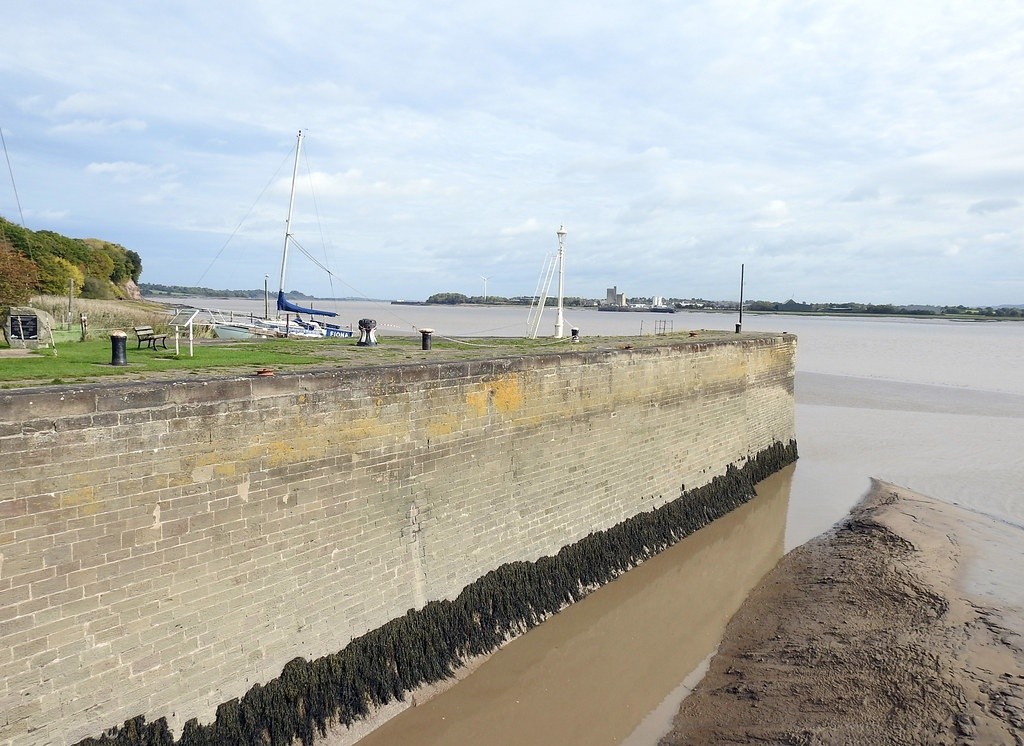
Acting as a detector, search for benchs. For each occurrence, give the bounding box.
[134,325,168,351]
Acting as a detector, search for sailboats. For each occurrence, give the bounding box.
[206,129,357,339]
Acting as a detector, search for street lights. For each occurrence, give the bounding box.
[264,274,270,319]
[554,224,569,339]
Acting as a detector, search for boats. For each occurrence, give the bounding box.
[598,303,675,313]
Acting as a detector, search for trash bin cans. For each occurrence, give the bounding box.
[355,319,378,347]
